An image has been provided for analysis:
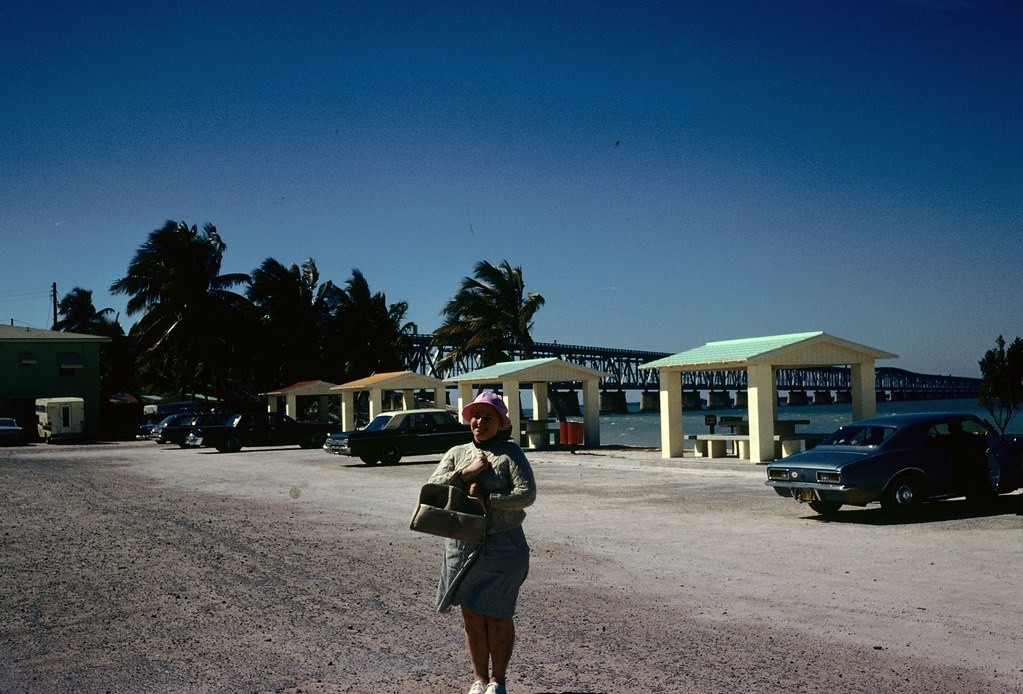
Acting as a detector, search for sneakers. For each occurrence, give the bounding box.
[468,680,507,694]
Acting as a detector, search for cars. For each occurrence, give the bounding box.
[764,413,1023,523]
[0,418,23,445]
[135,400,231,450]
[322,408,514,466]
[186,412,342,453]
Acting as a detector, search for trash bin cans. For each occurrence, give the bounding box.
[560,422,568,444]
[568,421,584,444]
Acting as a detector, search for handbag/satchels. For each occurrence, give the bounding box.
[409,470,490,546]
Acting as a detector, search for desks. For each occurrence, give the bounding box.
[718,419,810,456]
[520,419,556,448]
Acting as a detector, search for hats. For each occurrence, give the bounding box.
[462,391,512,429]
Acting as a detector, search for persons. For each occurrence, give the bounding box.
[427,392,536,694]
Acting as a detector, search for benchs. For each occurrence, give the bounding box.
[521,429,561,447]
[684,434,832,462]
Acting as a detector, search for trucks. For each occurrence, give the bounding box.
[31,397,85,444]
